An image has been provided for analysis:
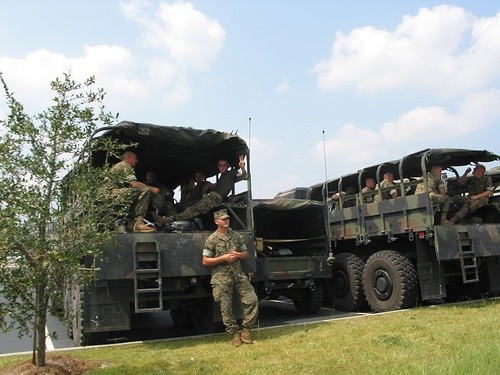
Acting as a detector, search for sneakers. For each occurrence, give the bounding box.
[241,328,253,343]
[133,219,156,233]
[232,333,241,346]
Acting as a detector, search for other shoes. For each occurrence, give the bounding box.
[154,214,172,225]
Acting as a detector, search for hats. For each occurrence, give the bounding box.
[214,209,230,219]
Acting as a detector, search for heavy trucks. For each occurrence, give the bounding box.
[50,120,257,349]
[274,146,500,315]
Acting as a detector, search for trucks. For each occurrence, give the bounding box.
[252,198,333,316]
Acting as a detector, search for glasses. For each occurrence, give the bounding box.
[219,164,227,166]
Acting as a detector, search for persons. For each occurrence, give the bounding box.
[331,166,493,225]
[98,152,246,233]
[202,209,258,346]
